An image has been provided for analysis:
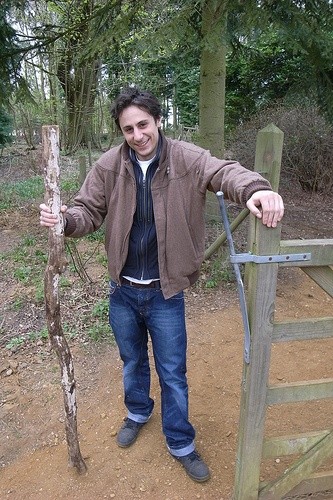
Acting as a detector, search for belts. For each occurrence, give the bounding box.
[121,278,161,288]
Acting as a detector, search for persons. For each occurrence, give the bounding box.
[40,87,285,483]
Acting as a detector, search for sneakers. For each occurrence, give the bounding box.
[116,417,147,448]
[169,448,210,483]
